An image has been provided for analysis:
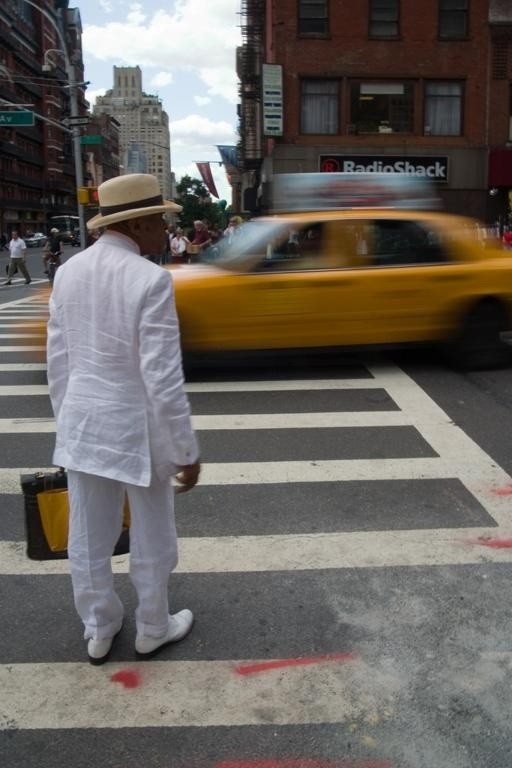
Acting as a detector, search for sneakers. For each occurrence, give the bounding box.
[25,280,31,284]
[45,270,49,274]
[4,282,11,285]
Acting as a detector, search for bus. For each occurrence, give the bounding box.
[50,215,80,238]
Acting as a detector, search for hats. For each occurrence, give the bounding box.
[86,173,183,229]
[51,228,59,233]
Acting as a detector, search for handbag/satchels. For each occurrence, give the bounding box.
[21,471,129,560]
[6,264,18,275]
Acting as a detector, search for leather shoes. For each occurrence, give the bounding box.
[135,608,193,658]
[87,639,114,665]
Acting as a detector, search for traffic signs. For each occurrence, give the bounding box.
[62,116,89,127]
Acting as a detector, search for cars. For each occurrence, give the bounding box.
[23,232,48,247]
[161,209,512,368]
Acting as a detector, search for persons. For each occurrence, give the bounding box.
[47,171,208,663]
[43,229,62,275]
[88,227,102,245]
[162,214,245,263]
[284,225,319,256]
[2,230,31,286]
